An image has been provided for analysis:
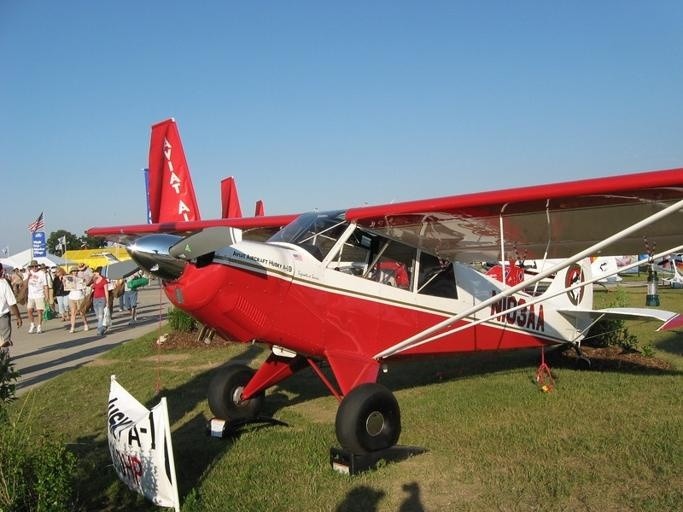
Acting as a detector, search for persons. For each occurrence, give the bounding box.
[0,261,22,350]
[0,264,162,337]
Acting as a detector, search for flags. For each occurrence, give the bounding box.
[24,210,45,233]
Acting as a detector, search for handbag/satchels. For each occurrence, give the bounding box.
[127,274,149,290]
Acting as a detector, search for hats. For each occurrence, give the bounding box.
[69,262,86,273]
[39,263,49,269]
[28,260,40,266]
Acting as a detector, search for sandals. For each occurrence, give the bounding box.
[79,296,92,313]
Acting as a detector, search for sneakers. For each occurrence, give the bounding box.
[37,327,41,334]
[27,326,36,333]
